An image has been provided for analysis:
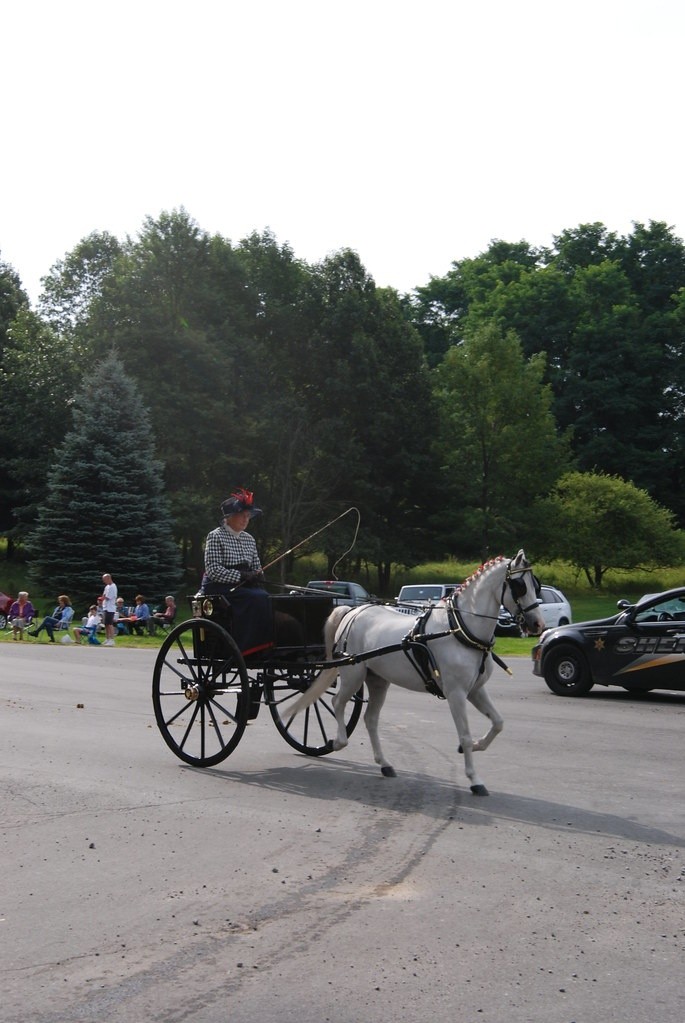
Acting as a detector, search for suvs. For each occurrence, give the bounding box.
[494,585,572,636]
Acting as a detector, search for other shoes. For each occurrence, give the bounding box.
[101,639,115,646]
[27,630,39,638]
[48,639,55,642]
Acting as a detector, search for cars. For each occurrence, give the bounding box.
[305,581,375,607]
[532,586,685,698]
[0,592,15,630]
[395,584,459,610]
[632,592,685,618]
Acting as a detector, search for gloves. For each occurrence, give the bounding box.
[240,570,262,583]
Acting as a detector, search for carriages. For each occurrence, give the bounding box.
[151,550,546,796]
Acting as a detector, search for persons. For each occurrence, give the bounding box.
[146,596,175,637]
[200,488,273,659]
[73,597,105,644]
[113,595,149,636]
[102,574,117,646]
[9,592,35,640]
[28,596,73,642]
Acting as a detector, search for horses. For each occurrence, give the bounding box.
[276,549,544,797]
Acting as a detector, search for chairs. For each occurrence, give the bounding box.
[201,571,225,597]
[155,607,177,638]
[40,609,74,642]
[78,622,99,645]
[142,604,152,636]
[126,607,134,617]
[5,612,32,642]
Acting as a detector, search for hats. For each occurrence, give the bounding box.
[220,488,262,521]
